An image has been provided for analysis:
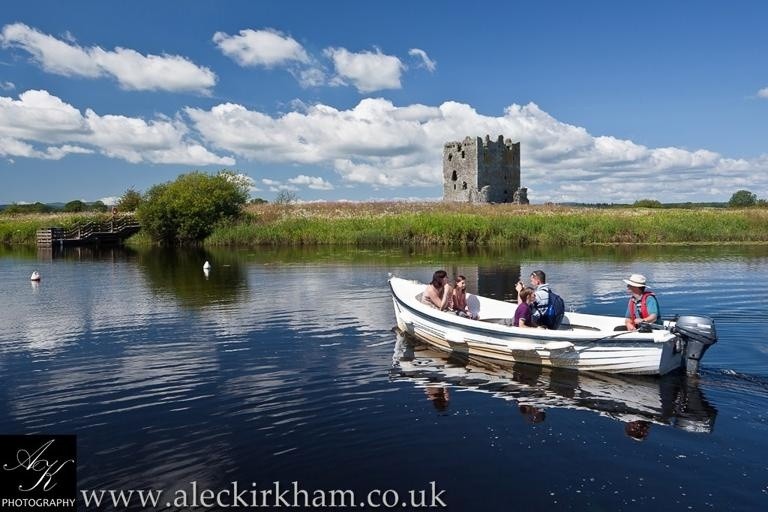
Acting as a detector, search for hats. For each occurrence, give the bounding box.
[623,273,652,289]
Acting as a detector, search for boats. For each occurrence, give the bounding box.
[387,270,718,377]
[385,342,721,454]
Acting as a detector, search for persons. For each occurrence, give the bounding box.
[610,273,660,332]
[423,381,450,410]
[512,287,550,330]
[450,276,475,320]
[513,268,551,328]
[518,401,548,425]
[625,421,655,442]
[421,270,454,313]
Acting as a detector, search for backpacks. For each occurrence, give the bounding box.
[534,288,564,330]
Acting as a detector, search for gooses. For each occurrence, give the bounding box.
[203,260,211,269]
[30,270,41,281]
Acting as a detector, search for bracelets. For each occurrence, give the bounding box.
[462,304,467,309]
[642,318,647,322]
